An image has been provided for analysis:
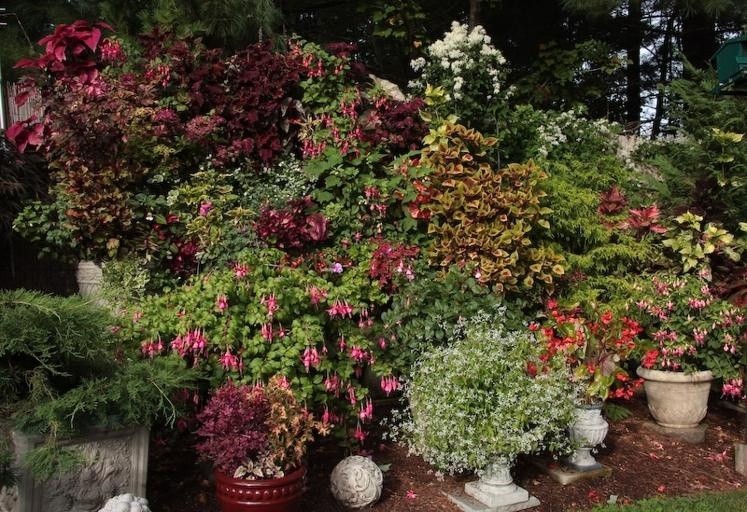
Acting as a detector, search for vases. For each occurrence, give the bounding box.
[215,466,307,512]
